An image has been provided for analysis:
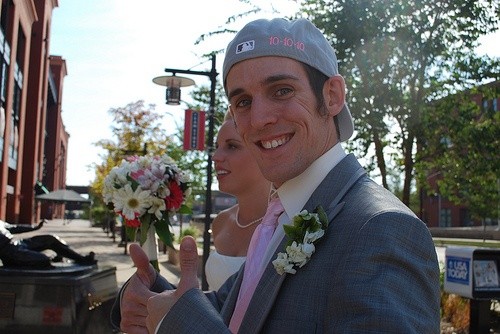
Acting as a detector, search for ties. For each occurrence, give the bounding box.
[228,191,285,334]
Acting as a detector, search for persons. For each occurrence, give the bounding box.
[205,108,270,291]
[0,220,96,268]
[110,19,441,334]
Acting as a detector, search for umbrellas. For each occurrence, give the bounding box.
[35,189,90,205]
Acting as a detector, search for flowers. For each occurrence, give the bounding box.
[102,153,193,274]
[271,204,329,274]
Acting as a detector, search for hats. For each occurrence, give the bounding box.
[222,18,355,142]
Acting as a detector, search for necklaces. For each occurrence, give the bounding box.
[236,207,264,228]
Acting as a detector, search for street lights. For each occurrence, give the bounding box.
[151,55,220,292]
